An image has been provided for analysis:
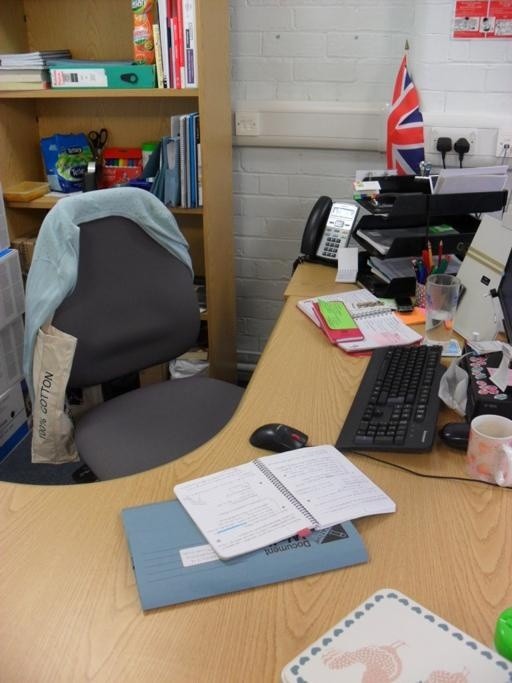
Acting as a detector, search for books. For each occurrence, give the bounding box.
[434,165,509,195]
[296,287,424,356]
[145,114,202,210]
[173,445,398,565]
[0,0,198,88]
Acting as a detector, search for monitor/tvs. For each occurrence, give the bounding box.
[437,247,512,450]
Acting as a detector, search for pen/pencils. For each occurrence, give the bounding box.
[412,240,450,284]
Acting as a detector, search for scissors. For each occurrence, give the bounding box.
[88,128,108,166]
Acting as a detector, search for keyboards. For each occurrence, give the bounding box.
[336,342,447,457]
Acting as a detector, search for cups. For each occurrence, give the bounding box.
[426,274,461,346]
[466,414,512,487]
[416,280,426,308]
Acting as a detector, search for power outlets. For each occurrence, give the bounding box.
[431,125,482,157]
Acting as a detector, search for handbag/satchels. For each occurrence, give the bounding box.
[30,325,80,465]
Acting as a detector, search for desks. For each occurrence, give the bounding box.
[0,242,512,683]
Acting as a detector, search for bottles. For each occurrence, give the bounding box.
[131,0,156,63]
[141,142,157,172]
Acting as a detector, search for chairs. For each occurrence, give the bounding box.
[25,184,247,479]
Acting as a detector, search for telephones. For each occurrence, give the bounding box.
[300,196,360,266]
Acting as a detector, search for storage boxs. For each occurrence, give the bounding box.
[0,181,30,467]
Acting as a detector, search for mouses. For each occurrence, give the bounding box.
[248,422,308,453]
